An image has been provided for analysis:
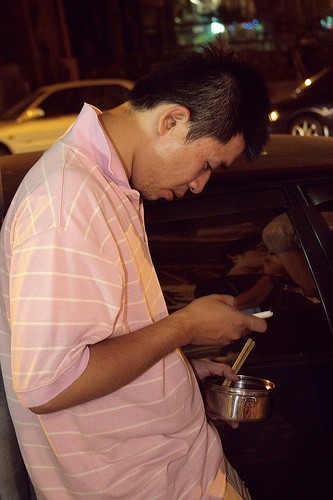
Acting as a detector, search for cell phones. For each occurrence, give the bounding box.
[253,311,274,319]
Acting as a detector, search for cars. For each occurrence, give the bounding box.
[0,78,136,157]
[146,162,333,500]
[0,135,333,500]
[269,67,333,138]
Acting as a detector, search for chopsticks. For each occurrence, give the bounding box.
[223,338,255,387]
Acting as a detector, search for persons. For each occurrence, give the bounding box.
[193,237,333,366]
[0,43,271,500]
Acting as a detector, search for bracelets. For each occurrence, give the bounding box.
[263,273,280,285]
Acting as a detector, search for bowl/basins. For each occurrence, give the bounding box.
[203,375,275,422]
